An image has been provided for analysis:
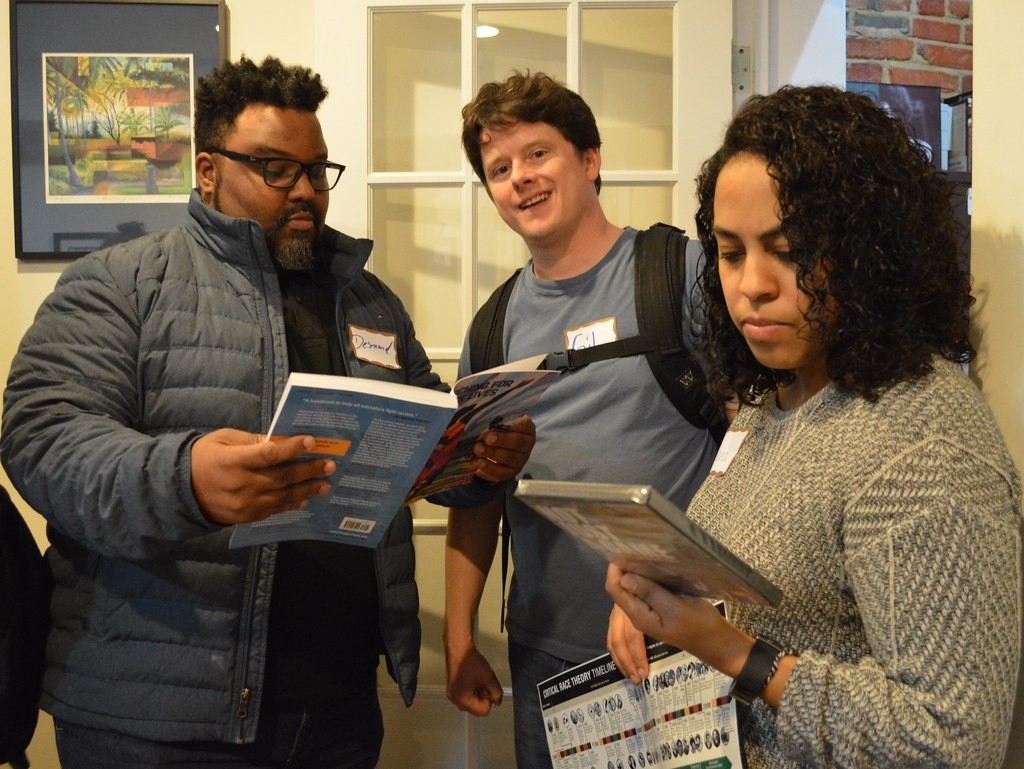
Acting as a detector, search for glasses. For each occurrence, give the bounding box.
[201,146,346,193]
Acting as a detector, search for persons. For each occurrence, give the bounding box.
[444,70,739,769]
[605,84,1024,769]
[0,57,536,769]
[605,660,728,769]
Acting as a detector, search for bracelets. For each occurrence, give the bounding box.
[725,633,780,707]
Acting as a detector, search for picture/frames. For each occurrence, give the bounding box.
[9,0,228,261]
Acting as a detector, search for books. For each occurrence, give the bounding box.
[228,354,561,550]
